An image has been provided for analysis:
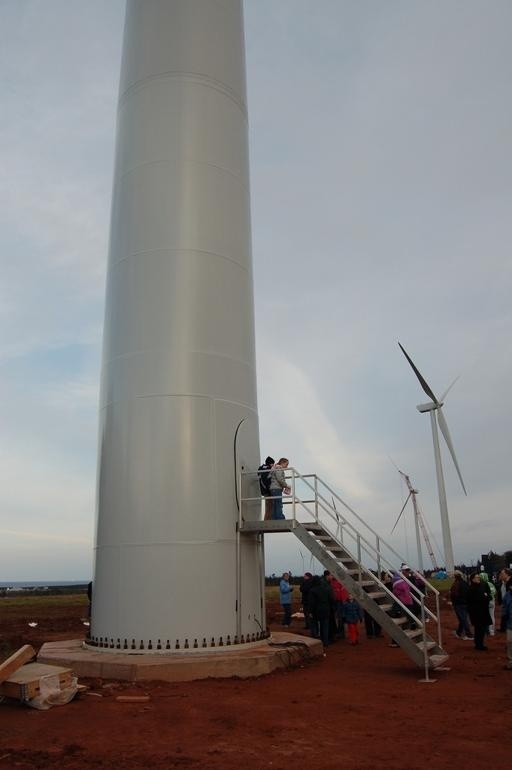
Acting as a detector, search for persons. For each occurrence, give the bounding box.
[299,563,428,649]
[257,456,275,520]
[449,564,511,671]
[279,572,294,630]
[268,457,292,520]
[86,580,93,619]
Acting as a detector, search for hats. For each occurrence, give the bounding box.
[266,457,274,465]
[401,565,411,570]
[392,573,401,582]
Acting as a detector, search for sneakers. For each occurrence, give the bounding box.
[456,630,487,650]
